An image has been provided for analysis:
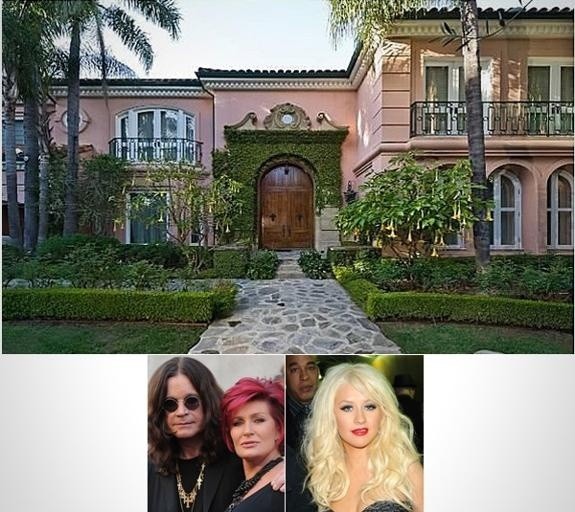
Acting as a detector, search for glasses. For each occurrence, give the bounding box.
[164,396,200,412]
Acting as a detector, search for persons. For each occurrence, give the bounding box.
[148,356,284,511]
[287,355,424,512]
